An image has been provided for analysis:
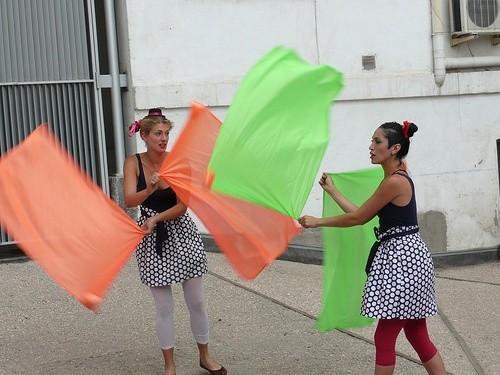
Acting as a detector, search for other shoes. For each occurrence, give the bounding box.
[198,357,228,375]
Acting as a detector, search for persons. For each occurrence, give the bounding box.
[124,109,228,375]
[299,121,448,375]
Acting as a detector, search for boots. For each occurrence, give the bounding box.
[425,351,447,375]
[374,363,395,375]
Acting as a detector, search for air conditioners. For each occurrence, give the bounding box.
[449,0,500,34]
